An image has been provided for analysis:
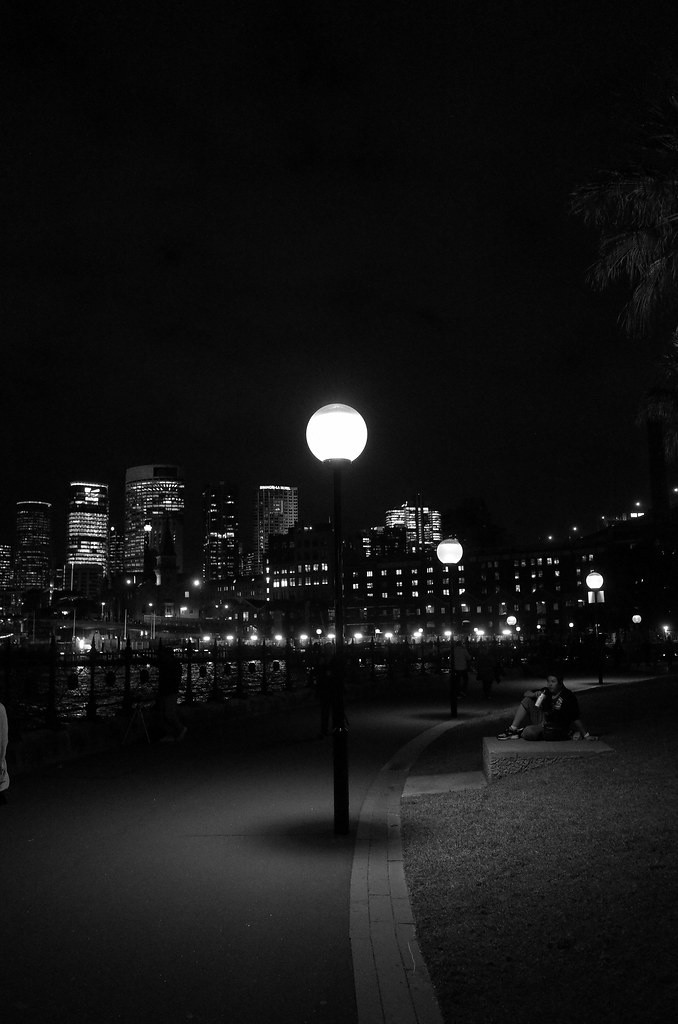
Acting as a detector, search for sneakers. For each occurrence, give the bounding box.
[498,727,525,740]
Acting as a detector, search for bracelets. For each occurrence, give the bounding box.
[584,732,590,738]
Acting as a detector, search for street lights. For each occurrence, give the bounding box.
[505,615,519,668]
[586,571,605,685]
[632,614,642,667]
[435,536,464,718]
[303,402,369,836]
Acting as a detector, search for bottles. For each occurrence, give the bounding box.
[535,690,546,708]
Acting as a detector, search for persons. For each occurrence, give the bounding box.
[307,644,350,738]
[496,671,598,742]
[452,638,501,701]
[157,647,188,742]
